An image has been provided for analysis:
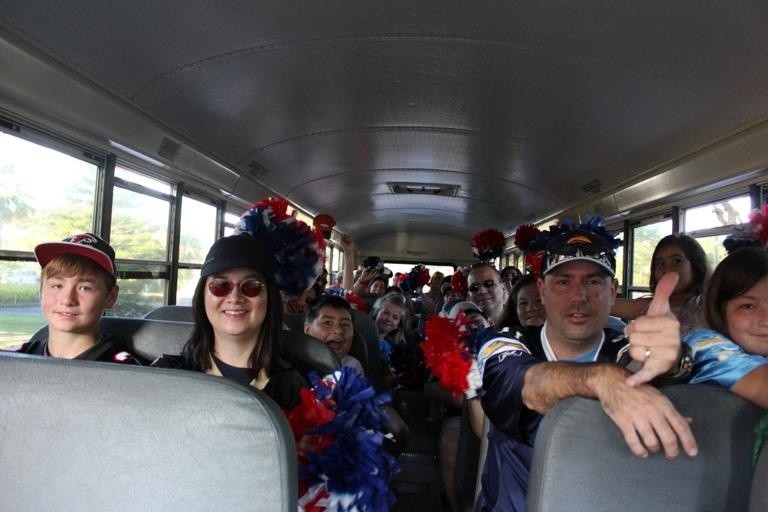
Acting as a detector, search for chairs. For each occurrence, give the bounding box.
[474,413,490,510]
[526,380,763,512]
[352,308,385,386]
[140,304,302,331]
[1,348,299,512]
[27,315,341,370]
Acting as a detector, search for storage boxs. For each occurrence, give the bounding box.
[749,437,767,512]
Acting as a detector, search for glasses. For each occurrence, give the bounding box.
[545,243,609,256]
[206,277,265,297]
[468,280,494,291]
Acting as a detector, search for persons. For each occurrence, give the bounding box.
[683,245,767,411]
[169,233,309,411]
[609,232,710,340]
[471,228,699,512]
[282,255,547,510]
[14,232,141,366]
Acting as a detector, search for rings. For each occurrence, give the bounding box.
[645,347,651,361]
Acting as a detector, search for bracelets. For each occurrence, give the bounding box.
[663,342,694,383]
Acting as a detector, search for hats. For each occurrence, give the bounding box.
[34,233,117,279]
[202,236,270,278]
[447,301,488,325]
[541,229,616,277]
[363,257,393,277]
[440,282,455,296]
